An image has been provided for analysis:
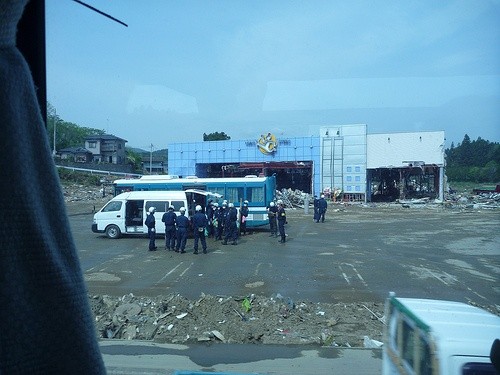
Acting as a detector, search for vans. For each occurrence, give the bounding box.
[89,188,225,239]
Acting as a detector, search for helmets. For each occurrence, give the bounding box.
[270,202,275,207]
[321,195,325,199]
[148,207,156,212]
[207,199,234,208]
[244,200,249,204]
[276,200,283,205]
[178,206,186,212]
[196,205,202,211]
[167,205,175,210]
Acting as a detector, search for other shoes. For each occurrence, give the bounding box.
[316,220,319,223]
[322,220,324,222]
[274,234,277,238]
[269,234,273,237]
[149,235,237,254]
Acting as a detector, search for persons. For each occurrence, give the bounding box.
[145,207,157,251]
[162,195,327,255]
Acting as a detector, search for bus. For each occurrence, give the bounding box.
[112,173,278,233]
